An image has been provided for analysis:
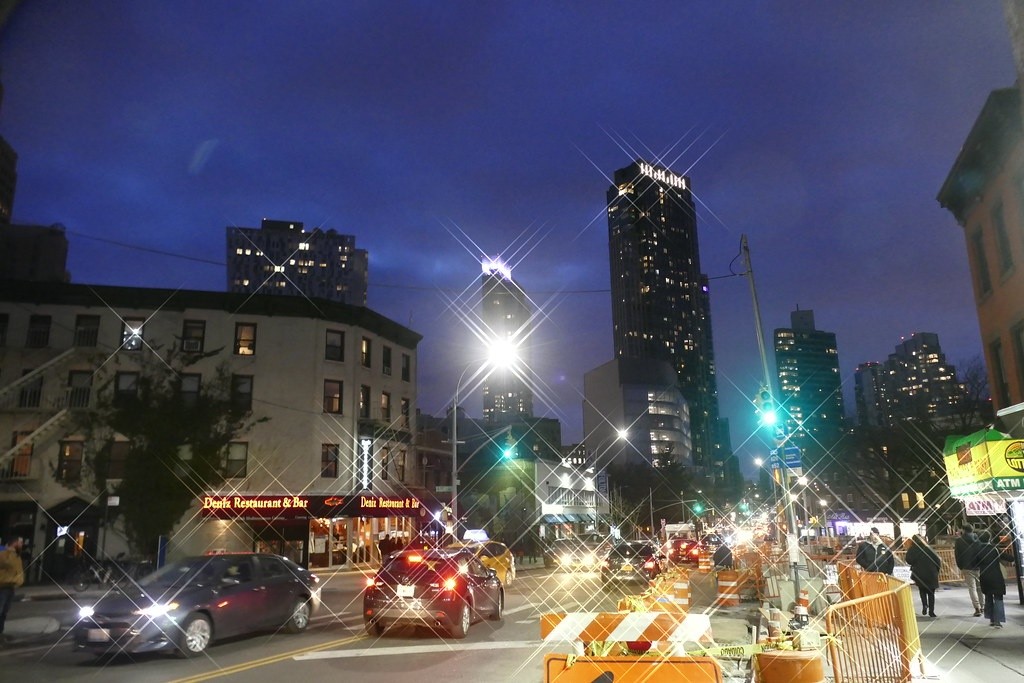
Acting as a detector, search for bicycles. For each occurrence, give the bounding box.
[72,551,132,593]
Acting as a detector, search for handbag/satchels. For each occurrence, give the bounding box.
[994,546,1009,580]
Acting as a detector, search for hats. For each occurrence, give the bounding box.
[871,528,879,535]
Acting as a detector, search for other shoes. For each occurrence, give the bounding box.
[929,612,938,617]
[0,632,16,642]
[990,623,994,627]
[980,609,984,614]
[922,605,928,615]
[973,611,982,616]
[994,623,1004,629]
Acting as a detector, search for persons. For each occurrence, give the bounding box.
[905,534,940,617]
[856,527,894,576]
[954,524,985,617]
[0,535,24,644]
[502,534,555,564]
[971,529,1014,629]
[379,534,404,563]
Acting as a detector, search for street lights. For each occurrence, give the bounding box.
[595,430,631,532]
[451,340,516,543]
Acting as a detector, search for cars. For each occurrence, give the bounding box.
[363,547,506,639]
[446,529,516,588]
[544,522,723,565]
[601,540,666,592]
[71,553,322,660]
